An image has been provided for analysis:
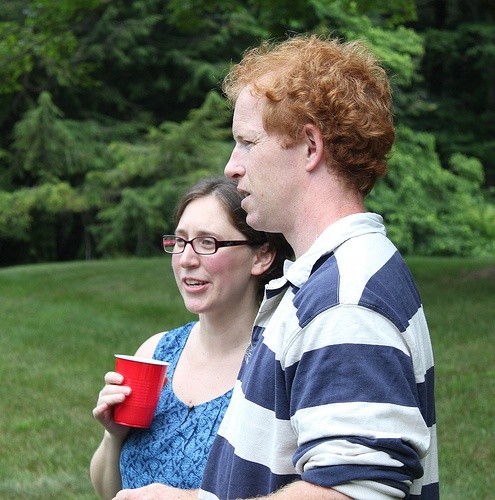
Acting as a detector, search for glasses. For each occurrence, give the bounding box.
[163,234,260,255]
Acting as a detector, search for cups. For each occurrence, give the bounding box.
[114,353,169,429]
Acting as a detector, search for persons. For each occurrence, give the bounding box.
[111,25,444,499]
[89,176,292,498]
